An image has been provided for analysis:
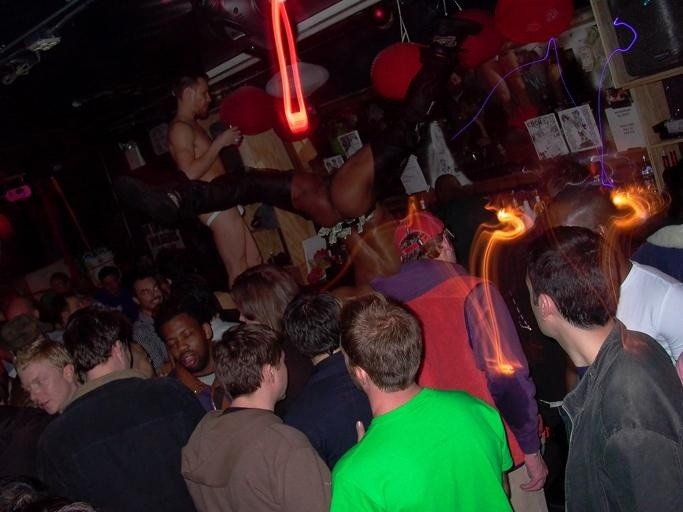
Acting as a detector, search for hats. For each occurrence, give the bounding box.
[393,212,455,256]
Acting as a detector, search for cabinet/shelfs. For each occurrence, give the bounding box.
[590,0,680,193]
